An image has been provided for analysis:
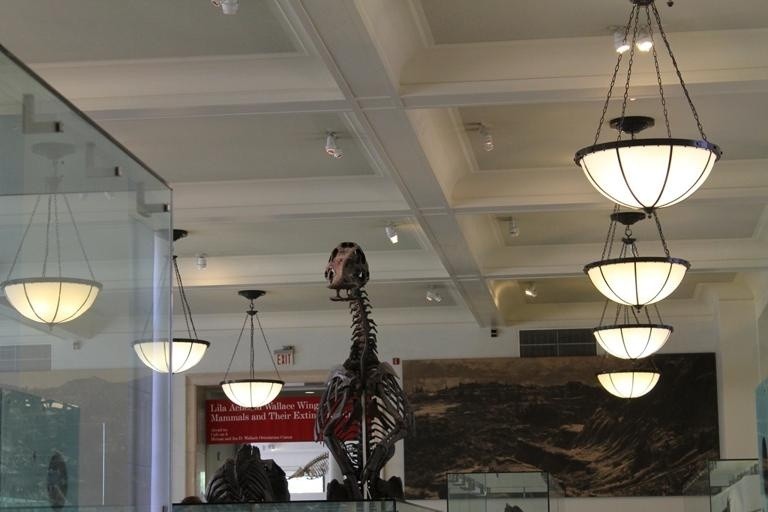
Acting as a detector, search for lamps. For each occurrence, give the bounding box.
[525,280,538,298]
[574,0,722,214]
[427,286,443,301]
[132,227,211,377]
[597,306,663,400]
[325,133,344,159]
[479,124,495,154]
[605,24,656,54]
[498,217,518,235]
[385,225,400,246]
[211,1,239,16]
[216,289,285,408]
[591,211,675,363]
[1,141,104,328]
[585,115,690,312]
